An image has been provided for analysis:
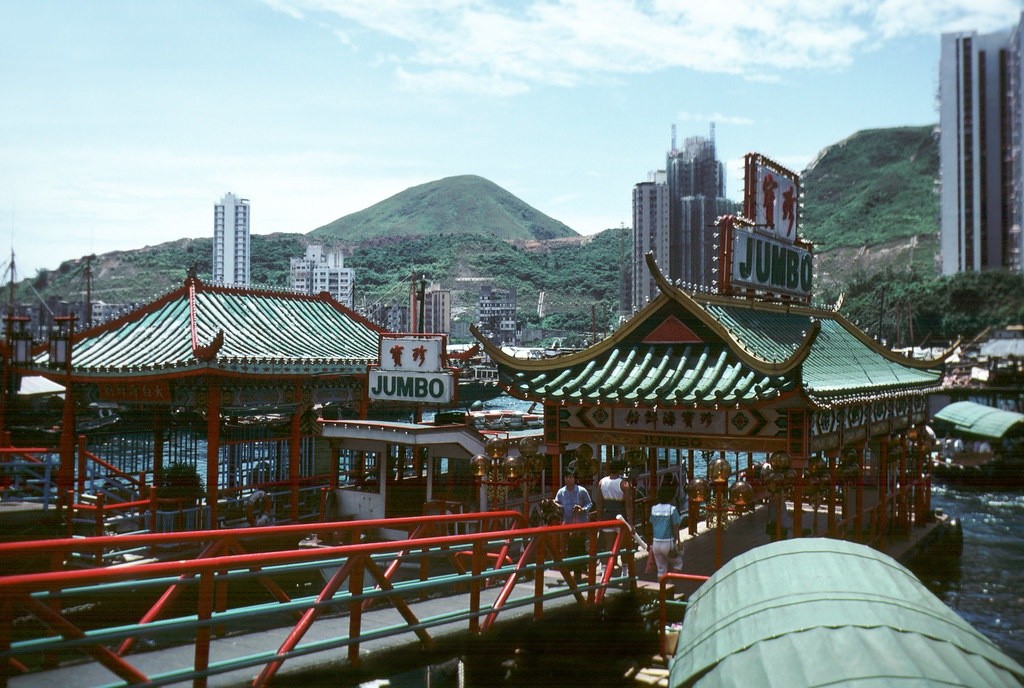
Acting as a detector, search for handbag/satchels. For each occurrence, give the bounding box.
[765,520,788,535]
[668,548,678,558]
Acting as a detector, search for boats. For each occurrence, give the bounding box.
[1,249,121,446]
[934,400,1024,489]
[472,410,546,450]
[455,361,503,404]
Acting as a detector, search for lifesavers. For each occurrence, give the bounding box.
[245,490,274,527]
[466,408,527,421]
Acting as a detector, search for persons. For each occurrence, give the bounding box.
[649,485,684,584]
[596,460,634,578]
[942,436,994,453]
[554,470,593,580]
